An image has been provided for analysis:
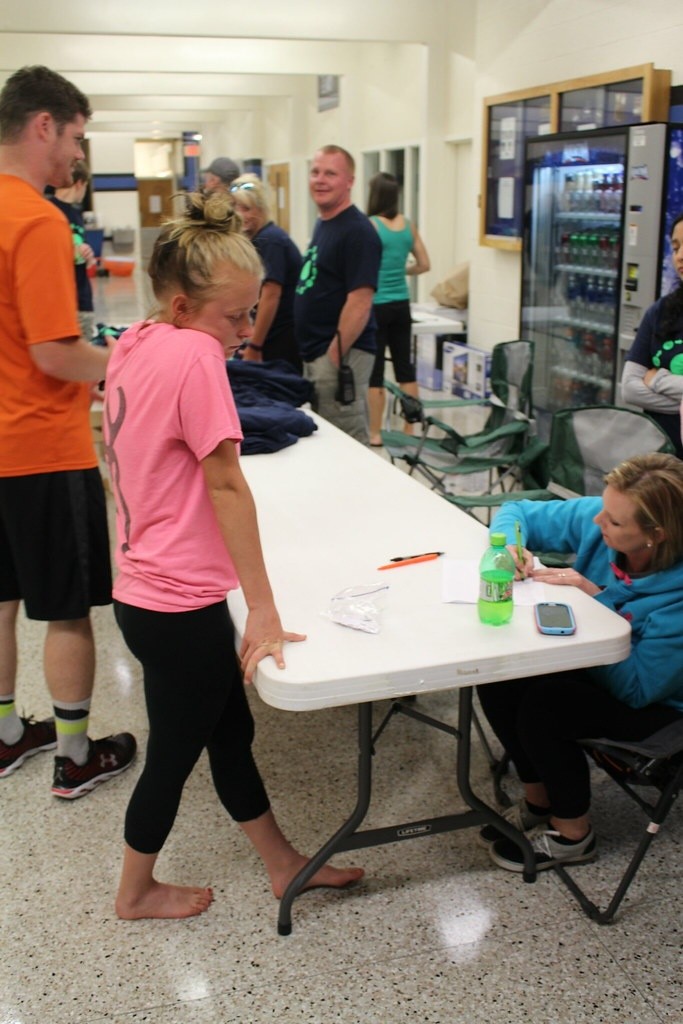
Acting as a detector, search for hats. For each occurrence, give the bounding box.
[199,157,240,181]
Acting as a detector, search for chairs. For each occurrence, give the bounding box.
[446,404,675,568]
[379,341,537,495]
[493,702,683,925]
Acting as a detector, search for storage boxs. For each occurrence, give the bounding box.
[410,311,493,406]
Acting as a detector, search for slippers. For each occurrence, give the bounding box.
[370,441,384,447]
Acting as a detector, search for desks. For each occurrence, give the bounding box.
[225,404,632,935]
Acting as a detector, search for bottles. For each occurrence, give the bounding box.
[567,273,617,329]
[560,173,624,213]
[549,328,616,414]
[553,220,621,271]
[478,534,516,628]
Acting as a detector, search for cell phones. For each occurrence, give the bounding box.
[534,602,576,634]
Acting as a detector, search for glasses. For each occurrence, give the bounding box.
[230,181,257,193]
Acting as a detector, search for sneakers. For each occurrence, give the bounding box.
[488,821,598,871]
[475,796,551,847]
[50,730,138,798]
[0,707,60,777]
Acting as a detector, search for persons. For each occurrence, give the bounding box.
[476,451,683,873]
[621,215,683,460]
[0,66,140,802]
[102,190,366,921]
[290,145,382,443]
[367,172,430,446]
[228,173,302,363]
[48,161,94,312]
[199,157,240,194]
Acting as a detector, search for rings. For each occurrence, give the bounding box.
[558,572,565,577]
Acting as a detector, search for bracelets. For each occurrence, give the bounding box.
[247,343,262,351]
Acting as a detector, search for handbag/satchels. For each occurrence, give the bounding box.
[225,341,317,454]
